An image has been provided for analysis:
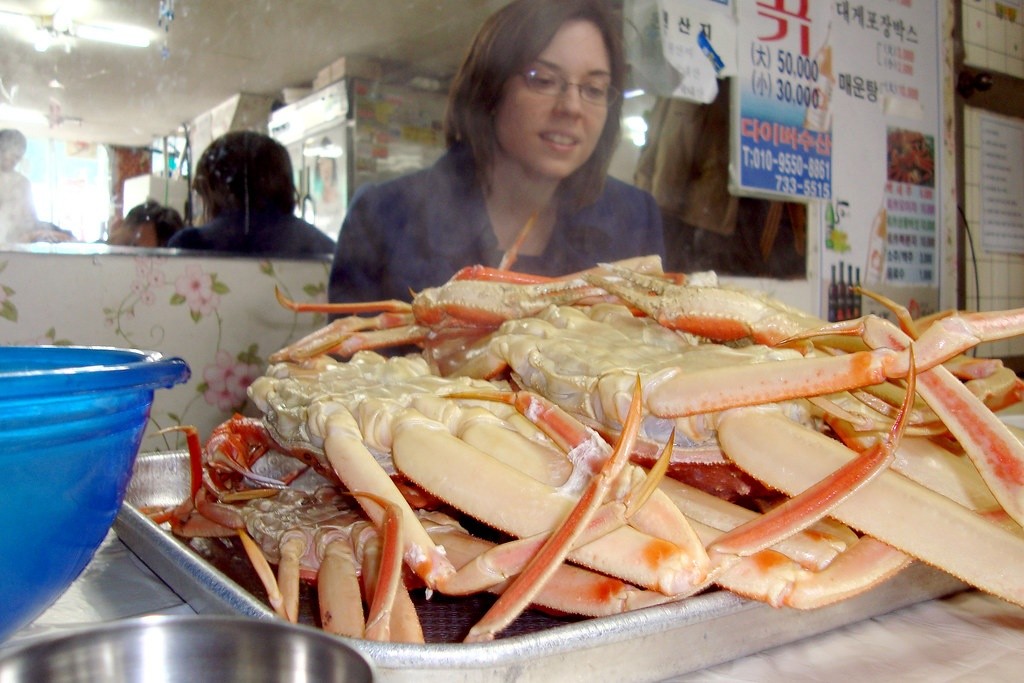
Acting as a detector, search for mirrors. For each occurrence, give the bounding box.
[0,0,730,262]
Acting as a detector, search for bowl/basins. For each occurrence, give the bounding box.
[0,343,193,645]
[0,616,379,683]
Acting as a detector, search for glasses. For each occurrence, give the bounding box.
[516,64,621,106]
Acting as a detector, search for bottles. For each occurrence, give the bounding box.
[805,24,834,131]
[828,262,861,321]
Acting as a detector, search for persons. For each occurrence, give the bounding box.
[169,131,335,255]
[0,129,73,243]
[106,199,184,247]
[328,1,669,363]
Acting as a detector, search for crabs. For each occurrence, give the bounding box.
[143,219,1024,643]
